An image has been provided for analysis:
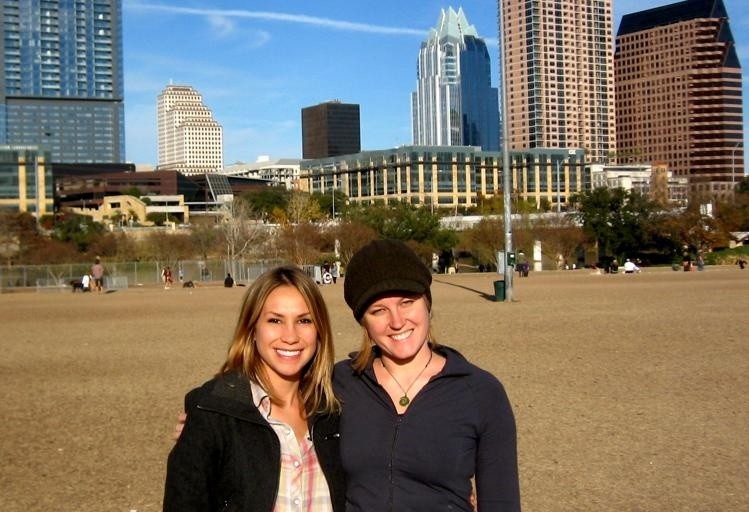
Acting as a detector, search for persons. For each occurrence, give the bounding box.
[160,266,172,290]
[679,244,706,272]
[604,258,643,274]
[163,265,343,512]
[81,272,90,293]
[323,258,338,285]
[515,249,531,277]
[735,257,748,270]
[173,237,523,512]
[92,258,104,296]
[225,273,234,288]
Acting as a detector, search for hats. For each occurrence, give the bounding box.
[344,240,432,320]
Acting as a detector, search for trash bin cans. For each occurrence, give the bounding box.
[494,280,504,300]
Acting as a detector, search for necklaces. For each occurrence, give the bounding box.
[379,348,433,407]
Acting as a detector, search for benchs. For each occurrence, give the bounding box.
[36,278,59,293]
